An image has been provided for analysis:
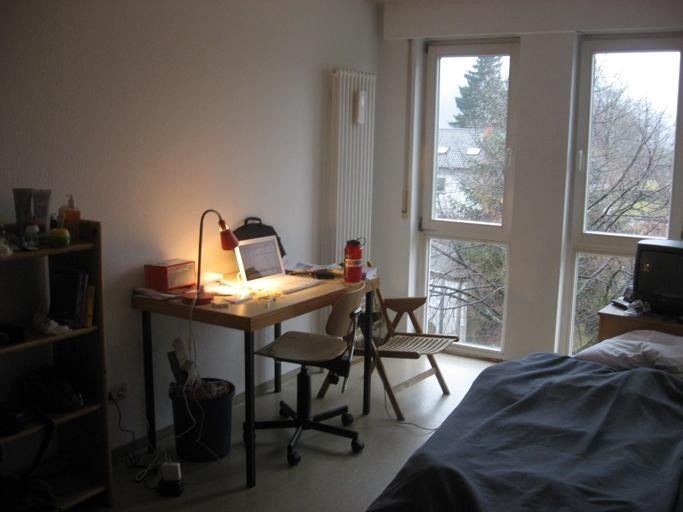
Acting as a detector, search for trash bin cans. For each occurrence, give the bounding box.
[168,378,235,463]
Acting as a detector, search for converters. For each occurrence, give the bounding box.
[160,462,182,481]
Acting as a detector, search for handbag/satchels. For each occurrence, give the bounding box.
[232,216,286,261]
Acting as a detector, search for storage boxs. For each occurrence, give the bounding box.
[143,257,197,291]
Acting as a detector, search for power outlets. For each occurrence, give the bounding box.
[105,382,128,406]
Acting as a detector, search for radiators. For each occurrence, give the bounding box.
[320,67,375,345]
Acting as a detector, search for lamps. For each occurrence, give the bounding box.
[184,209,239,304]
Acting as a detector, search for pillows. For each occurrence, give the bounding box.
[570,327,683,376]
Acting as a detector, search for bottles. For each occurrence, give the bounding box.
[342,237,366,282]
[23,219,40,248]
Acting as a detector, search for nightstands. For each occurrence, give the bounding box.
[596,292,682,344]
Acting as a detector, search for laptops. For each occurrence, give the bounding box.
[233,235,324,295]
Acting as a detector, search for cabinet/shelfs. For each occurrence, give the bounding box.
[0,220,111,512]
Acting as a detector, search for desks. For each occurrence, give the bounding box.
[133,269,369,490]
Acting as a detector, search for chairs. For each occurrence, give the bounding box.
[240,279,367,471]
[332,259,458,420]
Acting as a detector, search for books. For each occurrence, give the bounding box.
[284,260,337,276]
[44,258,98,330]
[362,266,377,279]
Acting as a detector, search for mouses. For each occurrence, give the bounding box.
[316,270,335,279]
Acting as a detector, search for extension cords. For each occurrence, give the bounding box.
[157,476,184,497]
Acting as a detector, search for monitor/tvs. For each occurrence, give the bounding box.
[632,237,683,320]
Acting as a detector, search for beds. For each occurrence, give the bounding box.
[365,355,682,512]
